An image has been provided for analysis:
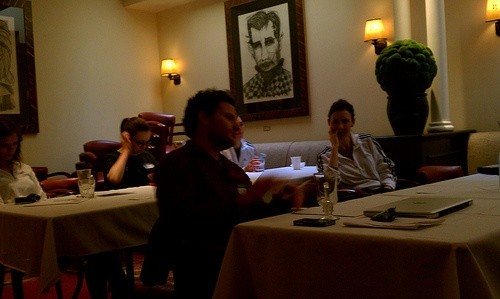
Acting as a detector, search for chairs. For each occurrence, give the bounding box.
[466,131,500,177]
[32,166,74,181]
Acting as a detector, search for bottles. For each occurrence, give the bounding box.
[254,153,266,172]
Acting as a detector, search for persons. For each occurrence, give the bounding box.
[0,118,131,299]
[102,117,155,190]
[317,99,397,195]
[156,90,302,299]
[223,116,260,171]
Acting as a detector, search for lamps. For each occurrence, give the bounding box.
[484,0,500,37]
[160,58,181,85]
[364,18,388,55]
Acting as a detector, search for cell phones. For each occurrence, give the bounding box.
[294,217,335,226]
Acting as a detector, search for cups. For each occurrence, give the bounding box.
[291,157,301,170]
[314,171,340,220]
[77,179,95,199]
[77,169,91,179]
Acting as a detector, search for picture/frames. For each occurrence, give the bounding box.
[0,0,40,135]
[224,0,311,120]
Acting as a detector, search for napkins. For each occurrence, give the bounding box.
[343,215,447,230]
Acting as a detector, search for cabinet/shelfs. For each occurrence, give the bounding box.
[375,129,475,187]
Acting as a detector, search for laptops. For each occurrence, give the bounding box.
[363,197,475,218]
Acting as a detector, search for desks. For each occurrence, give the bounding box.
[242,165,319,206]
[0,184,161,299]
[236,172,500,299]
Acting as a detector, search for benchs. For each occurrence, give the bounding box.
[244,139,333,171]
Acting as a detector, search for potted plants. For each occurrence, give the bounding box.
[375,39,438,136]
